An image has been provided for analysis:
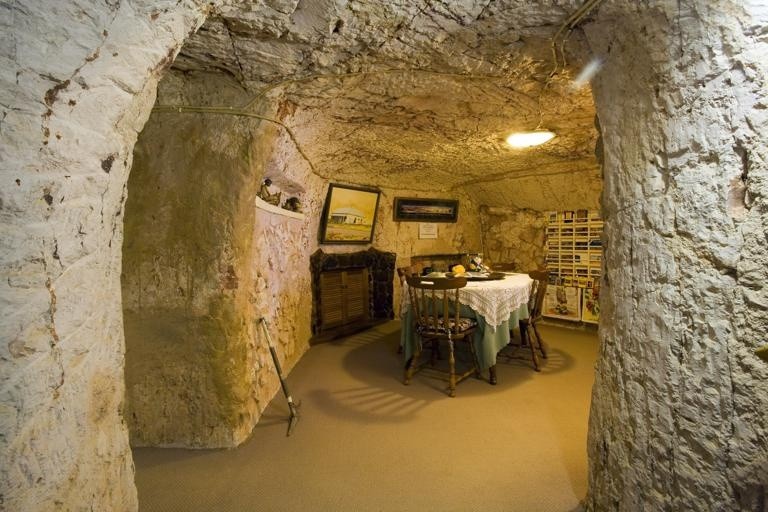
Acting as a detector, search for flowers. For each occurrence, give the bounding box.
[469,257,482,272]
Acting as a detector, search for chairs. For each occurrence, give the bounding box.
[404,277,481,397]
[520,270,550,371]
[397,265,439,353]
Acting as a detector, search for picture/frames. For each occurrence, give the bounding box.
[320,183,381,244]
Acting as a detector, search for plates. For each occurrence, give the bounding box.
[454,271,489,279]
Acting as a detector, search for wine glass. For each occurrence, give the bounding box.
[474,257,482,269]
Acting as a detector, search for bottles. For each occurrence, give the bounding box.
[465,253,470,272]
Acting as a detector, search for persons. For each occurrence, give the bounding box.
[258,178,282,206]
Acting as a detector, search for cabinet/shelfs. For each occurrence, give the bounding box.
[309,265,369,346]
[541,213,604,325]
